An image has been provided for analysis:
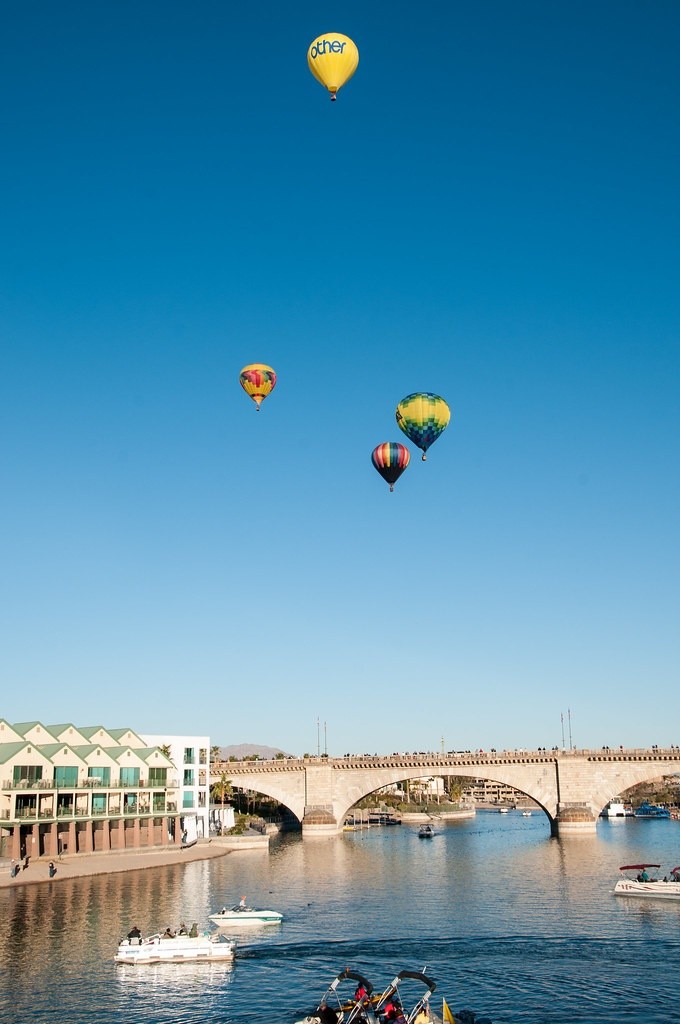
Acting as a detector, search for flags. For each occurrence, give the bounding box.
[443,998,455,1024]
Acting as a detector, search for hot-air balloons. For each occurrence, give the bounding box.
[239,363,277,412]
[370,441,411,492]
[395,391,451,461]
[305,32,359,102]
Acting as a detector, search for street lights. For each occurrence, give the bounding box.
[316,716,321,757]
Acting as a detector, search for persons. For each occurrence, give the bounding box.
[127,927,142,944]
[316,981,434,1024]
[10,860,15,877]
[426,826,431,831]
[49,860,55,877]
[180,924,188,935]
[240,896,246,906]
[637,870,649,882]
[164,928,176,938]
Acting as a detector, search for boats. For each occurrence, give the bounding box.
[418,823,436,838]
[365,813,402,824]
[293,965,492,1024]
[523,809,531,816]
[208,906,285,925]
[633,800,670,817]
[113,932,235,962]
[499,808,509,812]
[612,863,680,900]
